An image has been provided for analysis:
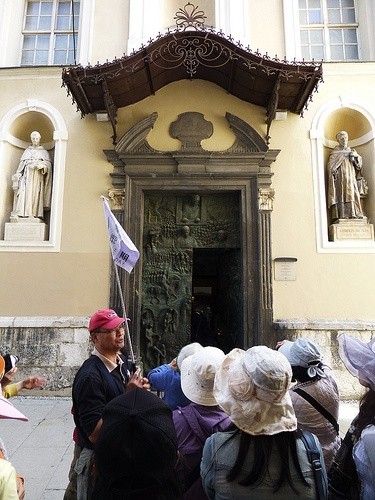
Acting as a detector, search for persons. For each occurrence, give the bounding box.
[88,330,375,500]
[62,308,150,500]
[327,130,369,220]
[0,353,49,500]
[12,130,52,220]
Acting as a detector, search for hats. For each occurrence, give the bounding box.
[176,342,203,371]
[0,355,5,377]
[0,383,28,422]
[87,309,131,332]
[94,387,177,495]
[277,337,327,378]
[180,346,227,407]
[213,346,299,436]
[336,333,375,391]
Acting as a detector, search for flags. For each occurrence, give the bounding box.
[104,201,141,274]
